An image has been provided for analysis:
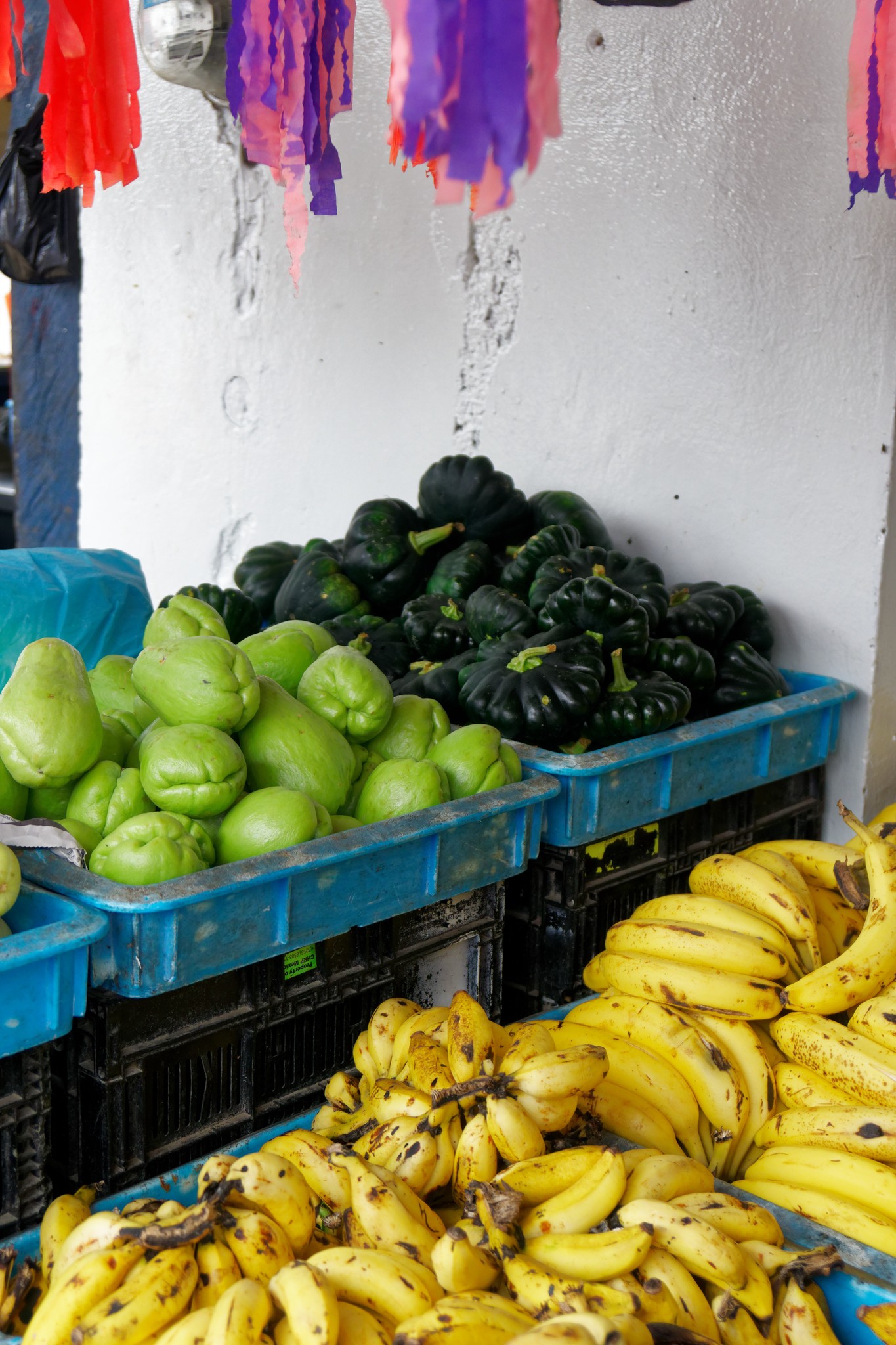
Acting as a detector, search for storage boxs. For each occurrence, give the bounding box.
[502,669,863,847]
[0,877,113,1060]
[504,764,831,1026]
[68,879,509,1204]
[0,754,561,1002]
[0,1023,85,1243]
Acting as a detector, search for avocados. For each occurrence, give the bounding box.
[1,593,522,895]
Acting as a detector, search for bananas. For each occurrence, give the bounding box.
[0,799,896,1345]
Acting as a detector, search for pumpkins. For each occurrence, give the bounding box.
[160,455,788,755]
